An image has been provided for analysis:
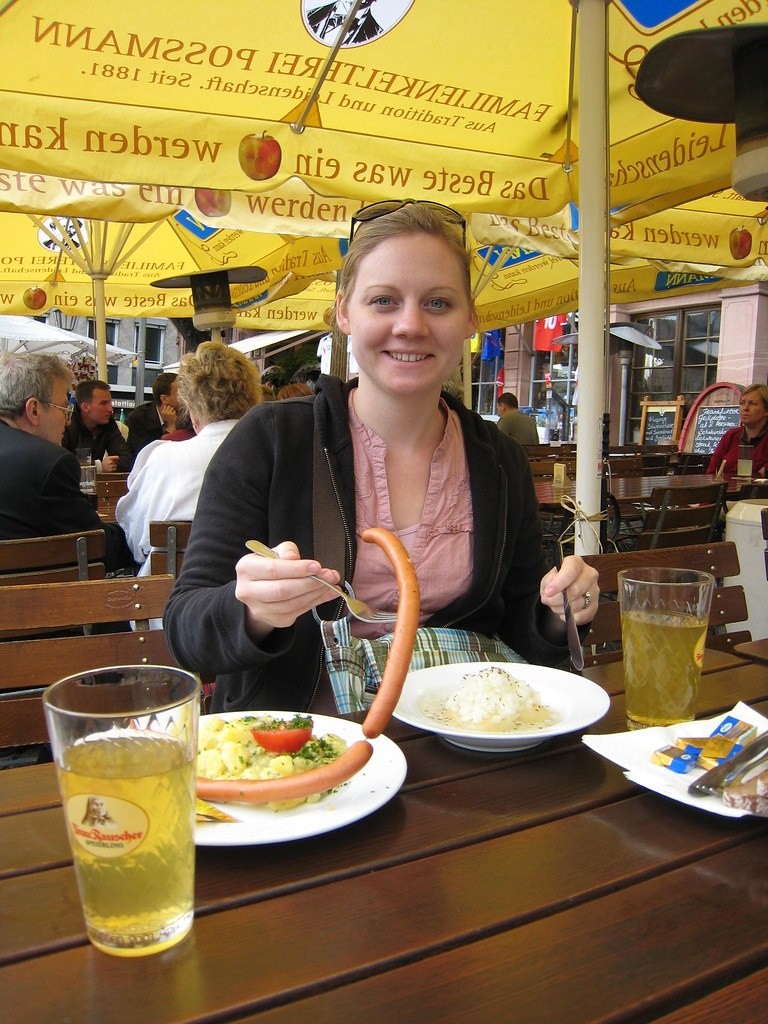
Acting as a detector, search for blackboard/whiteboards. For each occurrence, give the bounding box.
[642,411,676,445]
[692,405,741,454]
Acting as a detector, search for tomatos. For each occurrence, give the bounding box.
[251,717,314,754]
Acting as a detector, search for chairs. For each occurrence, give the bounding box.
[0,441,768,771]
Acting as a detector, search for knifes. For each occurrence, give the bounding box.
[554,542,586,672]
[687,734,768,798]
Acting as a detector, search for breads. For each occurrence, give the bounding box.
[722,768,768,814]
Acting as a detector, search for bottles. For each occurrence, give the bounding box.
[120,408,127,425]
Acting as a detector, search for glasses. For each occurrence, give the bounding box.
[23,396,74,425]
[349,198,466,253]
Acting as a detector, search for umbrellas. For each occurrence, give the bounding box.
[0,0,768,551]
[234,205,768,412]
[0,315,140,365]
[0,211,348,384]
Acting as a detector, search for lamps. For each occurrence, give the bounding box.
[632,21,768,204]
[150,264,269,332]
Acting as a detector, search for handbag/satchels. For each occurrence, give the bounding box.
[318,617,531,715]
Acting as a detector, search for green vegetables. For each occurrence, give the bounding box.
[226,712,348,795]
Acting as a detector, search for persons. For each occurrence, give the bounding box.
[128,372,181,453]
[261,364,321,400]
[62,380,133,474]
[115,342,264,634]
[497,392,539,445]
[698,384,768,507]
[164,203,599,717]
[0,351,136,685]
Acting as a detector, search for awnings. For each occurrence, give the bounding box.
[164,329,328,374]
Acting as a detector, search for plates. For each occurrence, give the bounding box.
[192,710,408,847]
[393,661,611,751]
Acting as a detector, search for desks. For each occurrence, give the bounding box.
[0,645,768,1024]
[533,475,764,570]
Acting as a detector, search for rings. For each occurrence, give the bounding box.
[583,592,591,609]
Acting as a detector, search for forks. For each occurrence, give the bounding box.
[245,540,398,624]
[696,730,768,796]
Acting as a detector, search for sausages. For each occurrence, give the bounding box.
[362,527,419,740]
[195,740,373,804]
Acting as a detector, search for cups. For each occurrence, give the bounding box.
[74,447,98,494]
[42,664,202,959]
[618,567,716,732]
[737,446,752,479]
